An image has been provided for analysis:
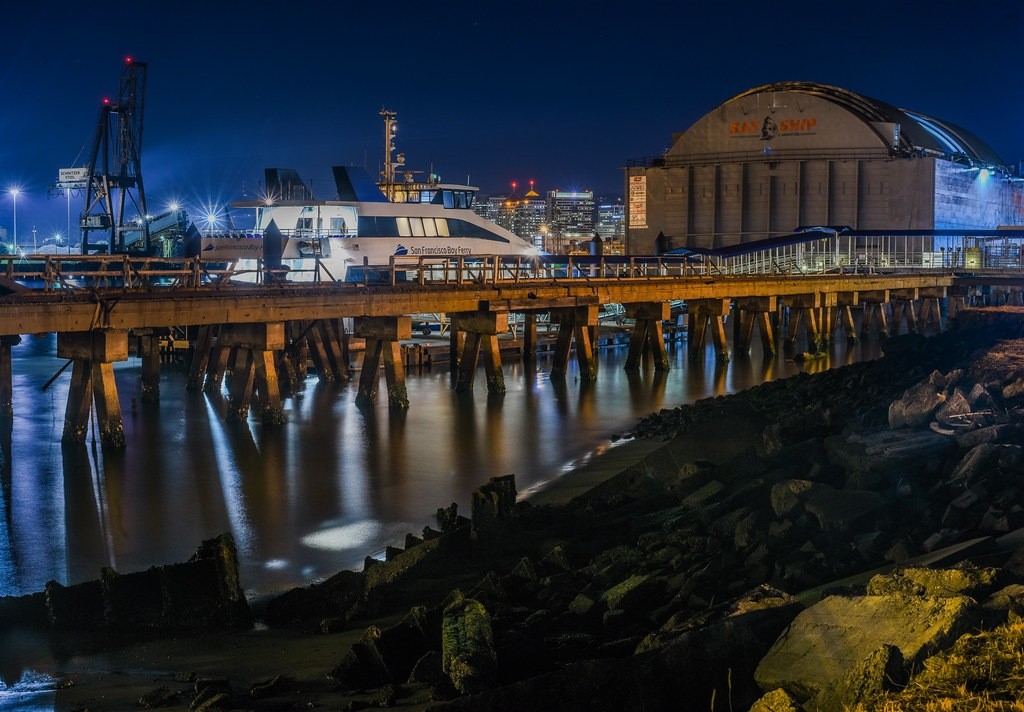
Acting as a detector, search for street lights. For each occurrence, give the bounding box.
[11,190,19,254]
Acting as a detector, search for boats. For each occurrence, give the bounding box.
[223,110,543,282]
